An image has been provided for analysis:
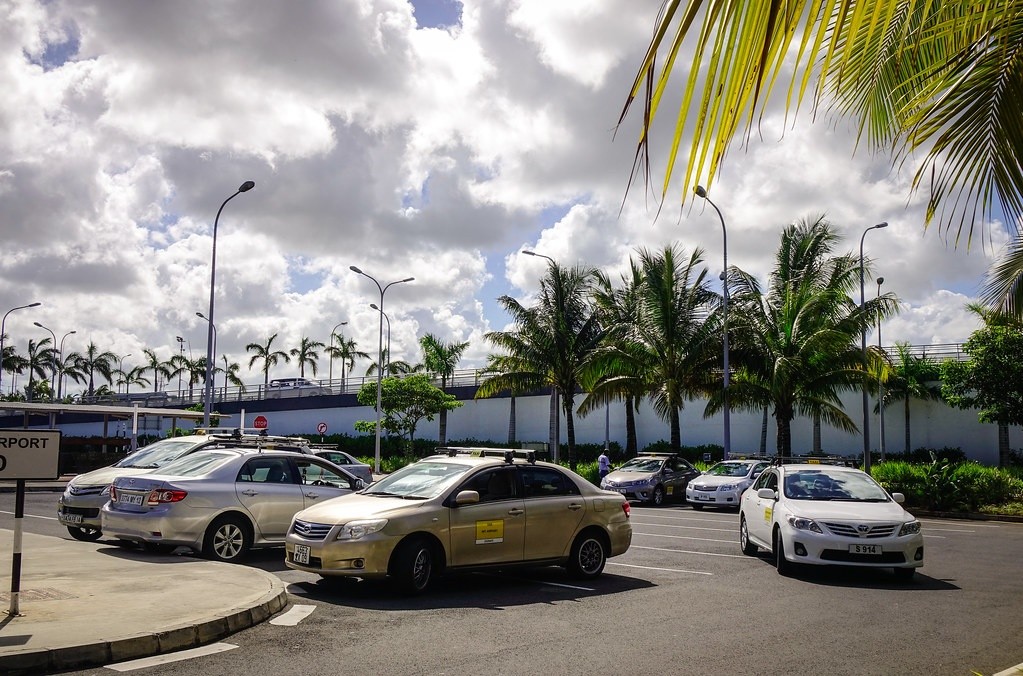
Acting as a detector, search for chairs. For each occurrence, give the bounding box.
[266,465,284,480]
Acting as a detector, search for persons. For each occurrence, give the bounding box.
[598,449,614,484]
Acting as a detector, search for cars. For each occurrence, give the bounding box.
[285,446,633,597]
[303,444,373,484]
[57,427,325,542]
[269,377,333,399]
[99,434,373,562]
[738,457,925,579]
[686,455,773,511]
[600,451,701,508]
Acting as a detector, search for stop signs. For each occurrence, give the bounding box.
[253,416,268,430]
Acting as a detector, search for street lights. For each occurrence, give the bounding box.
[520,249,561,465]
[196,312,217,433]
[58,331,78,399]
[348,265,415,475]
[858,221,889,471]
[118,354,133,394]
[33,321,57,404]
[0,302,42,390]
[692,183,731,461]
[202,180,257,436]
[875,276,888,465]
[329,322,348,390]
[370,303,391,379]
